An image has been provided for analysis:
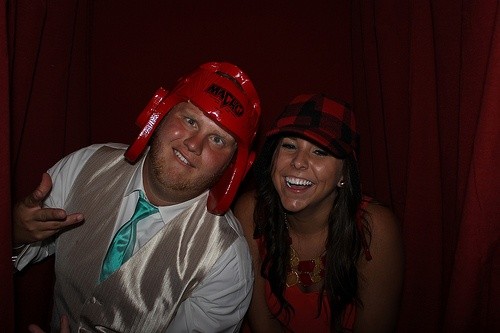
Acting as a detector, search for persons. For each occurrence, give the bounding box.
[231,92,400,333]
[10,61,261,332]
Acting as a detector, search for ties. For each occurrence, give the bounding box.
[100,190,159,283]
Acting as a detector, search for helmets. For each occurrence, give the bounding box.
[124,61,262,215]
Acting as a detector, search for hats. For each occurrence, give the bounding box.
[264,90,359,159]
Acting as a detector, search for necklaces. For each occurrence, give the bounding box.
[282,210,329,292]
